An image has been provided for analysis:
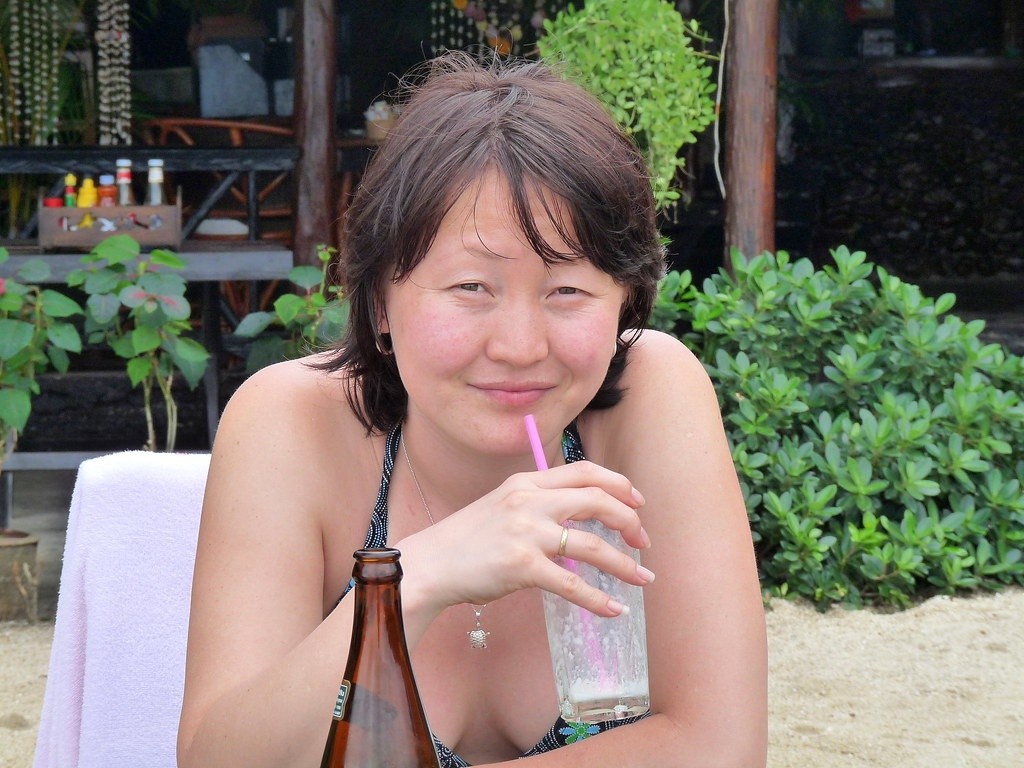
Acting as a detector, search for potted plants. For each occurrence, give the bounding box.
[0,247,85,629]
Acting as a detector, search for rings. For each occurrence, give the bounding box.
[559,527,568,556]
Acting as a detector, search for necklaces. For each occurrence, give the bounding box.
[400,422,490,649]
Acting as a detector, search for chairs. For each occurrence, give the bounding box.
[139,118,372,374]
[28,450,212,768]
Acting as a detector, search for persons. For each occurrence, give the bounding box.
[178,58,768,768]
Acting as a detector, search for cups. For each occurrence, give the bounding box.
[541,510,650,724]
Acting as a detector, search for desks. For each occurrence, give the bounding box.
[0,234,295,531]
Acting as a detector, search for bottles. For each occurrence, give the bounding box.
[115,157,139,229]
[44,169,117,232]
[141,159,168,229]
[320,548,440,768]
